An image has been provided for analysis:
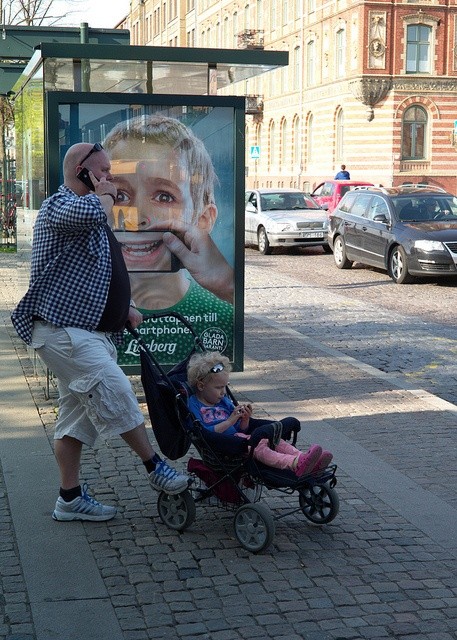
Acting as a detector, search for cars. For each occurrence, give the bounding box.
[327,184,457,284]
[245,187,334,254]
[305,180,375,216]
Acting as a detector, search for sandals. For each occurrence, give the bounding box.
[296,445,322,476]
[312,450,333,477]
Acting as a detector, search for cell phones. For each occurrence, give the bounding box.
[76,167,101,192]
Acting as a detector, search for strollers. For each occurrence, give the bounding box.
[125,312,339,553]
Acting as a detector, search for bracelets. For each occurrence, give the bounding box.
[129,304,136,308]
[100,191,116,206]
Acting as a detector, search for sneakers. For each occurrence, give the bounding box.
[52,483,117,521]
[147,455,195,495]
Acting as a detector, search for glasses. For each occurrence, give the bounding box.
[79,143,103,165]
[199,363,224,381]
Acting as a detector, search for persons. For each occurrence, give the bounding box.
[185,349,339,479]
[96,113,235,362]
[333,164,350,180]
[10,142,194,528]
